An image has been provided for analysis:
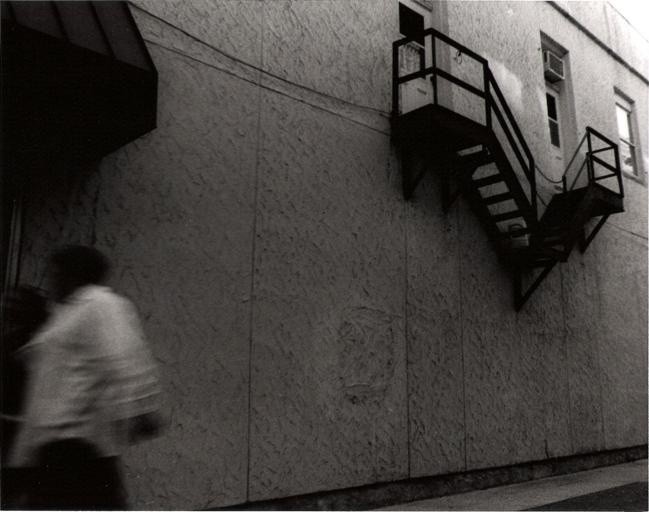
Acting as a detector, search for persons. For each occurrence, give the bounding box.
[2,233,160,510]
